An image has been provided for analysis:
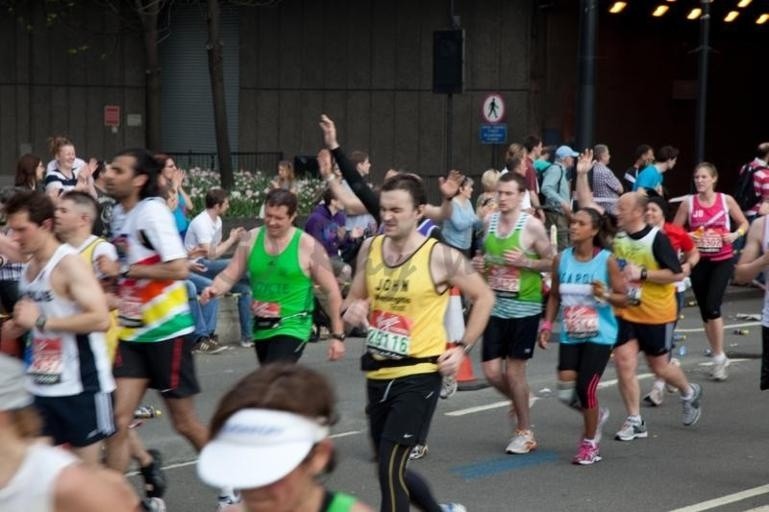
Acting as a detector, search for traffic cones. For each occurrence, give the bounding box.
[446,281,491,392]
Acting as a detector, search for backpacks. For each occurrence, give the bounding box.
[536,164,563,205]
[732,163,766,211]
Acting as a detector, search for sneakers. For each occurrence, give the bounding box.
[571,406,649,465]
[507,390,536,418]
[215,490,242,512]
[440,374,457,399]
[191,338,229,355]
[139,449,166,498]
[209,334,235,350]
[408,442,428,460]
[707,354,730,381]
[645,357,703,426]
[505,426,537,454]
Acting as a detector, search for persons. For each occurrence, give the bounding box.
[0,114,769,512]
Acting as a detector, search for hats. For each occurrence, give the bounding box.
[196,406,330,491]
[0,353,35,413]
[555,144,580,161]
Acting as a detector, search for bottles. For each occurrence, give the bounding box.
[694,226,705,238]
